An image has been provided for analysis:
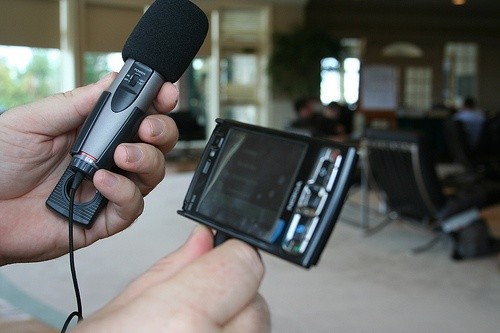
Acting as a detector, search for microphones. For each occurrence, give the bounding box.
[45,0,209,229]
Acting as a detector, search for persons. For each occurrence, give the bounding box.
[455,94,485,124]
[313,101,347,143]
[291,98,325,137]
[0,72,273,333]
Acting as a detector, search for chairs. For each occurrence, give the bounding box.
[367,142,500,262]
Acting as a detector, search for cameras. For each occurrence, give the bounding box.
[177,118,359,269]
